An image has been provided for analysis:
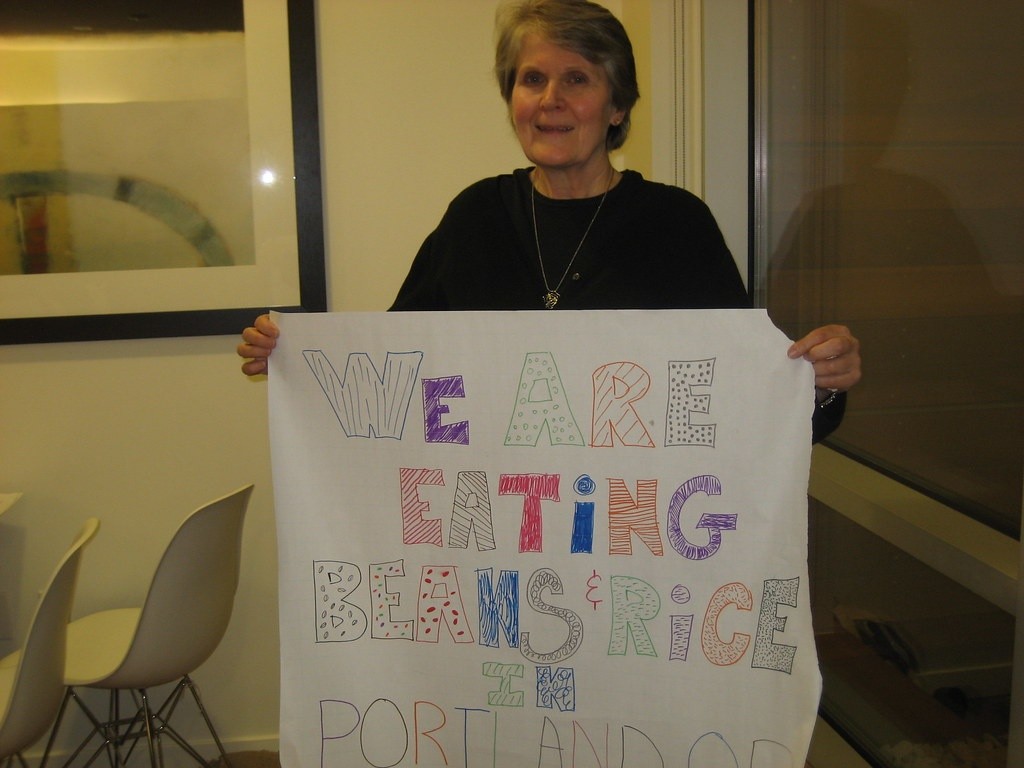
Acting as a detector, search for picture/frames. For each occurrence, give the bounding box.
[0,0,329,344]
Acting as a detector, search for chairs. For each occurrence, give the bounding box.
[0,476,117,768]
[61,483,252,767]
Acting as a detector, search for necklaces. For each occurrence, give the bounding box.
[531,165,614,309]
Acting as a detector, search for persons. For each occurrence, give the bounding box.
[237,0,863,444]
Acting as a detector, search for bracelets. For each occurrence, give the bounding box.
[818,392,836,408]
